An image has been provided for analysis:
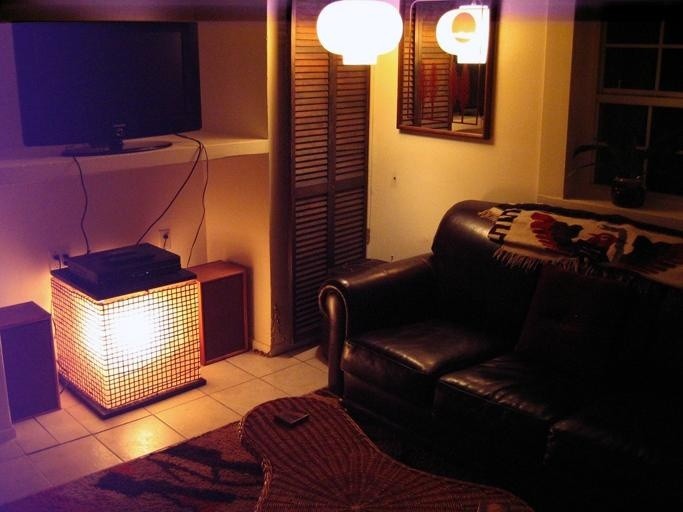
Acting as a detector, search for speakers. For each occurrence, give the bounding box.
[0,301,61,424]
[184,260,249,366]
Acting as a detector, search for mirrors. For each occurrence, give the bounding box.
[395,0,493,141]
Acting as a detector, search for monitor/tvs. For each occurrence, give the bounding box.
[12,20,202,157]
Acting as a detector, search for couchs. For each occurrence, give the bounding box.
[311,196,683,511]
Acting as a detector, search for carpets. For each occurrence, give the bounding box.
[2,382,594,510]
